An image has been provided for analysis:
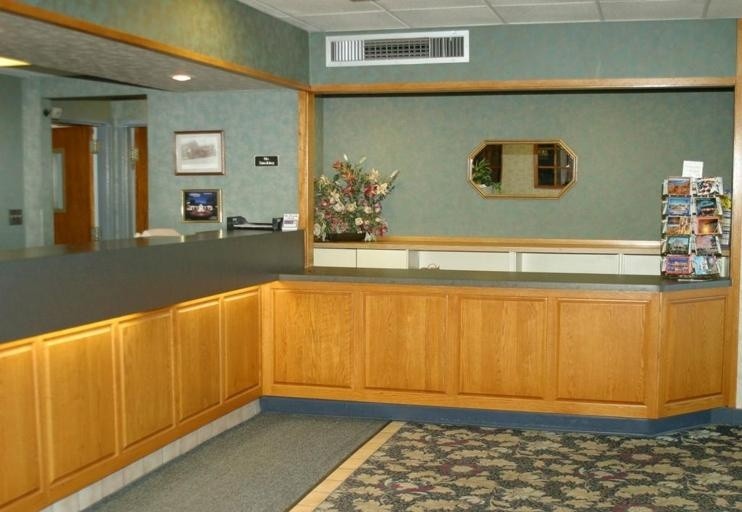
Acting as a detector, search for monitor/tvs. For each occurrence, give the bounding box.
[661,175,731,282]
